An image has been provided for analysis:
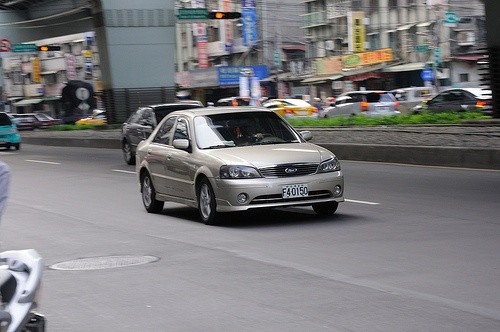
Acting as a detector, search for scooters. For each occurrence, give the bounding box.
[0,248,47,332]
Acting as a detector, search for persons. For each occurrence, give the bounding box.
[313,97,324,110]
[0,161,11,220]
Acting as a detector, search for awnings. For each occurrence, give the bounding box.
[300,74,344,84]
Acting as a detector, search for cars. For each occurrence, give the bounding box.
[0,111,22,151]
[216,94,320,119]
[75,111,107,125]
[120,103,205,165]
[391,87,438,117]
[134,106,345,226]
[319,90,401,120]
[8,112,63,131]
[407,88,493,117]
